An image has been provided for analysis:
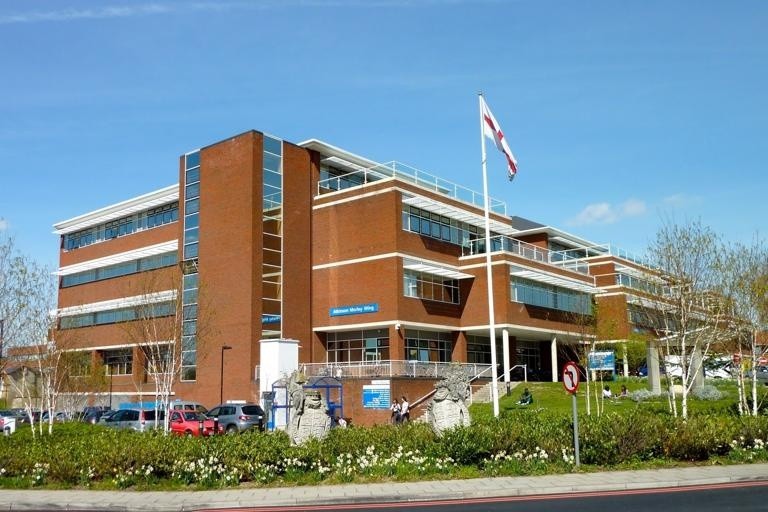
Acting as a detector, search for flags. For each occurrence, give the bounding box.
[478,87,518,182]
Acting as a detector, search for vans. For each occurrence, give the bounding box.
[206,403,266,434]
[170,399,208,415]
[104,409,166,433]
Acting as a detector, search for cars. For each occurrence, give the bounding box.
[169,409,223,437]
[745,365,768,386]
[1,400,115,433]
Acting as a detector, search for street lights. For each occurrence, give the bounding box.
[220,345,232,404]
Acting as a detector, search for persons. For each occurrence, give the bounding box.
[601,385,612,400]
[389,398,401,426]
[514,388,533,405]
[399,396,412,425]
[613,384,628,399]
